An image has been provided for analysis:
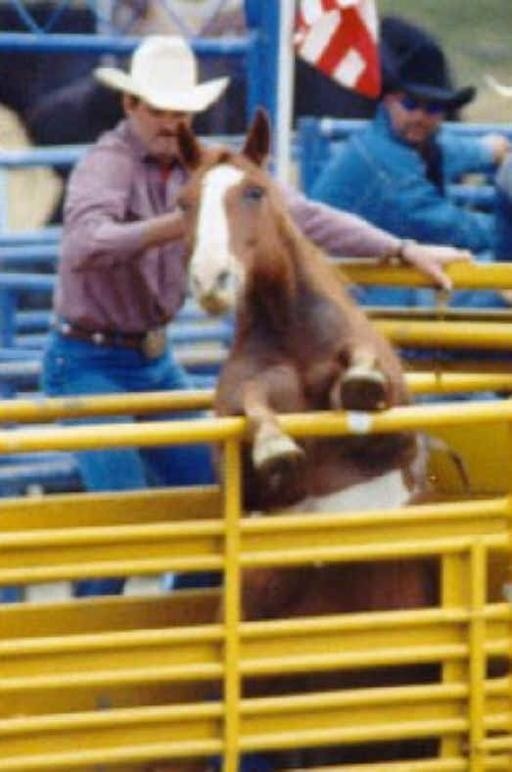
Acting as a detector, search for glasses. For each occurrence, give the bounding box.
[392,88,448,115]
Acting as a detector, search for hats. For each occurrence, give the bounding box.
[378,42,477,110]
[92,34,231,114]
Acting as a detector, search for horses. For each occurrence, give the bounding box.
[174,105,444,772]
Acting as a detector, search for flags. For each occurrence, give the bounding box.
[292,0,384,100]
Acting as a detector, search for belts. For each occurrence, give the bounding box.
[55,323,167,360]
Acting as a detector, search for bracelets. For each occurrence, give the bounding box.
[396,237,418,268]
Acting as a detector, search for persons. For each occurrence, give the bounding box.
[37,32,478,600]
[307,42,512,362]
